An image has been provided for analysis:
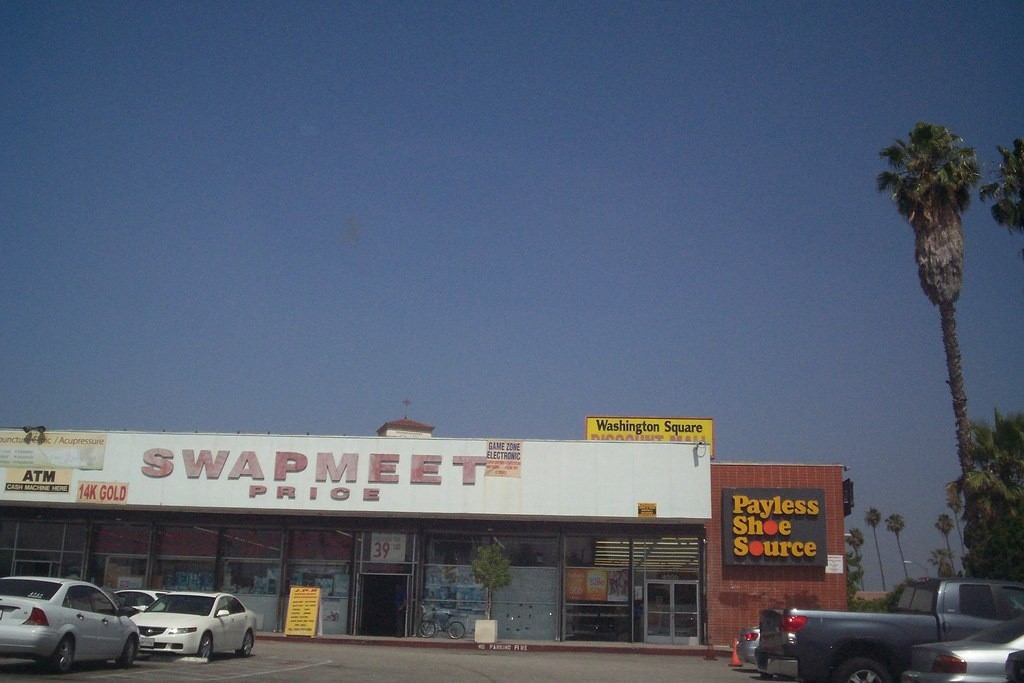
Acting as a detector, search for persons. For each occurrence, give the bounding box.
[394,580,407,638]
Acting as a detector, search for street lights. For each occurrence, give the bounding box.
[903,560,929,579]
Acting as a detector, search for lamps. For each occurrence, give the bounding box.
[35,426,46,433]
[23,426,34,433]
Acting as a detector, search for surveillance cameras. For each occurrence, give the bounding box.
[699,440,703,445]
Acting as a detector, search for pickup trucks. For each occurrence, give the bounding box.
[755,576,1024,683]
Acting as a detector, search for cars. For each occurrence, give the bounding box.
[114,589,166,611]
[902,616,1024,682]
[738,625,760,662]
[129,592,258,660]
[0,575,140,672]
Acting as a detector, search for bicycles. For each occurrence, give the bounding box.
[417,607,465,639]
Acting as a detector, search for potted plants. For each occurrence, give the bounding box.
[470,543,514,643]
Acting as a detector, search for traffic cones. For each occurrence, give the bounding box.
[703,636,719,660]
[728,637,743,666]
